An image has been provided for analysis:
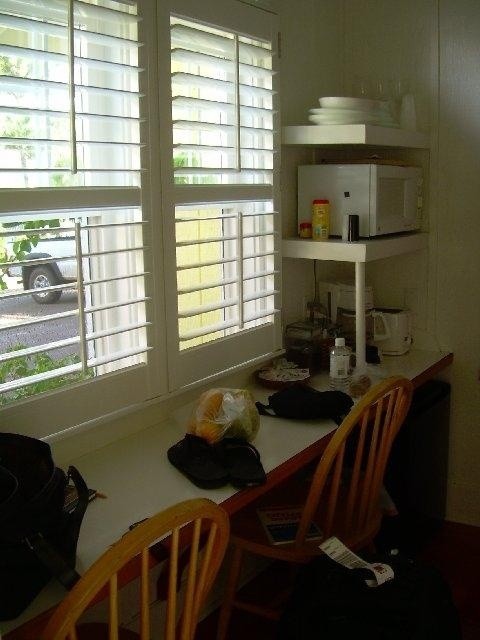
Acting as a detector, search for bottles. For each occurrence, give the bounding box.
[330,337,351,397]
[299,200,331,240]
[339,188,356,243]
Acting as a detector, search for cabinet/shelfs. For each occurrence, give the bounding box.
[282,108,428,372]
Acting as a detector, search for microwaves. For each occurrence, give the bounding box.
[297,164,424,242]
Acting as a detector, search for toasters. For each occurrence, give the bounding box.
[372,308,412,357]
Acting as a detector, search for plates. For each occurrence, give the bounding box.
[309,97,387,126]
[253,368,311,390]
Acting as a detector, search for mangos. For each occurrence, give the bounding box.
[191,387,224,441]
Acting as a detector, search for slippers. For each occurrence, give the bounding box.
[167,433,229,489]
[213,437,266,488]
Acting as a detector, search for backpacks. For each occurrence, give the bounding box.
[277,553,462,639]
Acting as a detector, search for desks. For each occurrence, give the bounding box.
[0,349,452,640]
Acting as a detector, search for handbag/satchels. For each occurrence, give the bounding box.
[255,381,361,439]
[0,432,89,624]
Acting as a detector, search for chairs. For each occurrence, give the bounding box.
[46,498,232,639]
[219,378,414,639]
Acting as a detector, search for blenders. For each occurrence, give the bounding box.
[320,275,377,357]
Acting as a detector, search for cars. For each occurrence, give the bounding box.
[22,231,81,304]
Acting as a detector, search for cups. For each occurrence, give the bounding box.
[399,92,417,134]
